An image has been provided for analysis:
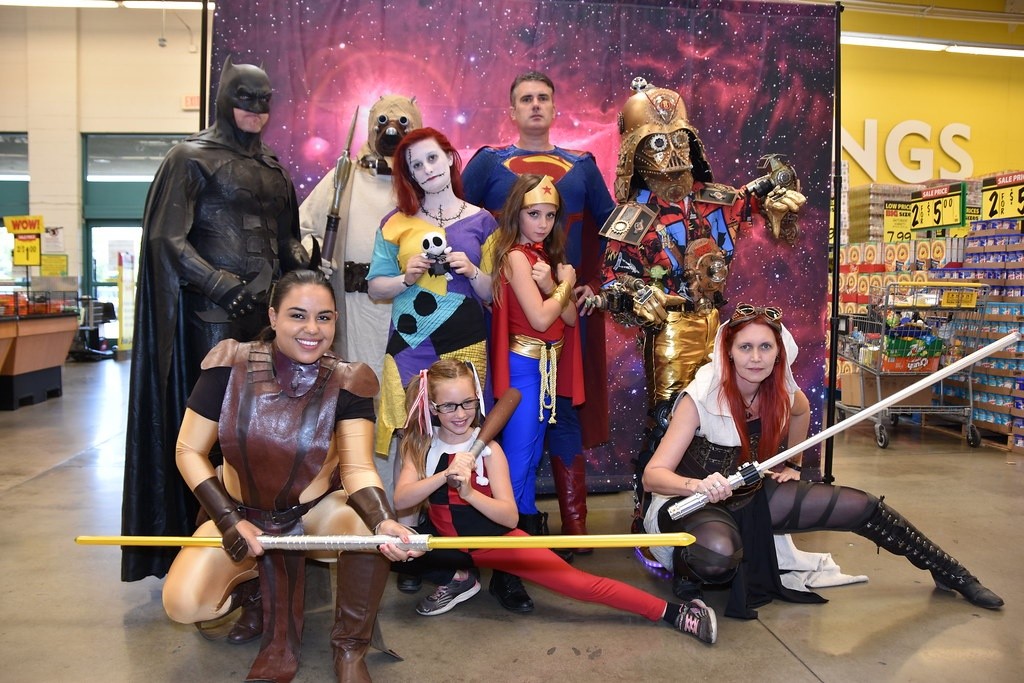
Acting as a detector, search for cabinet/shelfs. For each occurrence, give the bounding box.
[930,221,1024,450]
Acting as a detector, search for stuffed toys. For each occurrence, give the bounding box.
[422,232,453,282]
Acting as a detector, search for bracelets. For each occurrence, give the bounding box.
[786,460,805,474]
[468,267,478,281]
[401,280,416,287]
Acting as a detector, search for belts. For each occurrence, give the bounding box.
[219,476,335,526]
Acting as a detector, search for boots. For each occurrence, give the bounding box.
[330,549,391,683]
[852,495,1006,608]
[394,527,423,591]
[488,511,551,612]
[213,576,264,645]
[550,454,594,553]
[673,546,738,601]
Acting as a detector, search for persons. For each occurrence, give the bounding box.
[492,172,582,612]
[299,67,424,593]
[642,303,1005,621]
[163,269,423,682]
[121,54,321,584]
[601,76,806,581]
[458,73,616,557]
[392,358,719,647]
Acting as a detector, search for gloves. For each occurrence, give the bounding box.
[308,255,338,283]
[204,268,258,321]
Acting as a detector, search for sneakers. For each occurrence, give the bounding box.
[416,569,481,615]
[674,599,717,643]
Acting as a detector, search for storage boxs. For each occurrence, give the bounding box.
[840,339,942,408]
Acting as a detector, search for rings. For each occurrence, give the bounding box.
[585,293,596,308]
[705,485,732,491]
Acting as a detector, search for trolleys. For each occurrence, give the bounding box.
[835,282,990,450]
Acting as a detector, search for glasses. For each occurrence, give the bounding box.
[733,304,782,320]
[431,397,482,419]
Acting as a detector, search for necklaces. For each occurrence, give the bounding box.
[738,388,760,418]
